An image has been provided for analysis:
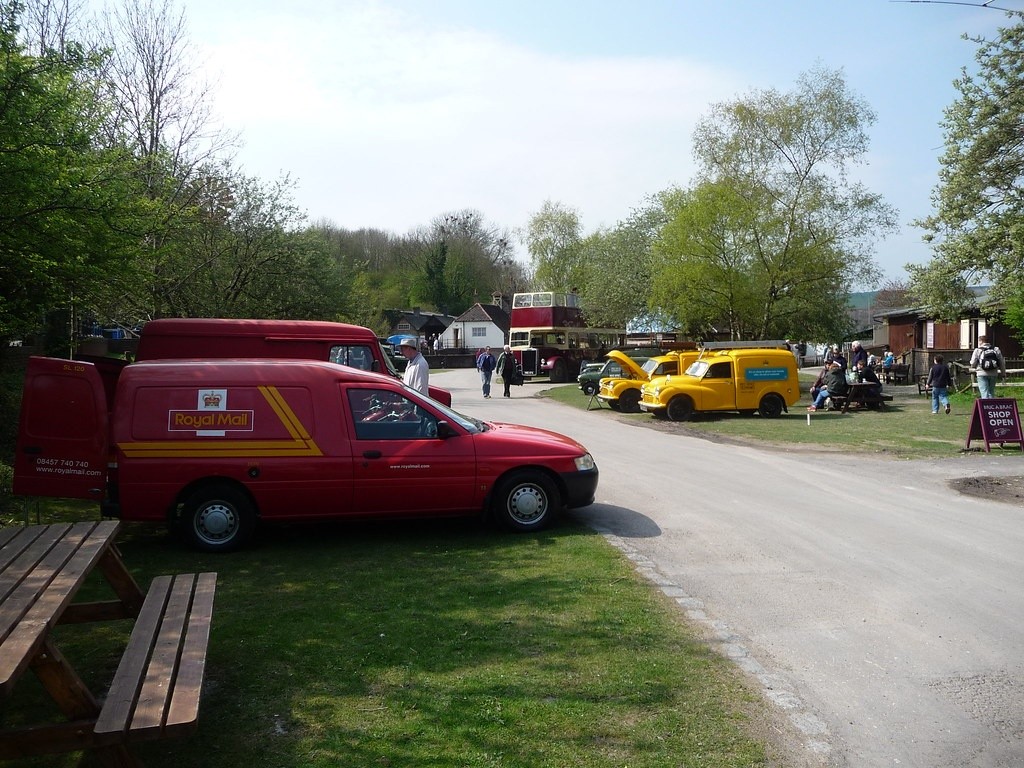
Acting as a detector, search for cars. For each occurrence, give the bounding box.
[577,348,665,397]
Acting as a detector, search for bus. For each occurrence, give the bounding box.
[505,291,627,384]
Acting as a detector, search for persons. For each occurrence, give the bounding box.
[970,335,1006,399]
[476,348,483,372]
[785,337,894,414]
[496,345,517,397]
[400,337,430,402]
[418,333,440,358]
[476,345,497,399]
[333,347,372,371]
[925,355,953,414]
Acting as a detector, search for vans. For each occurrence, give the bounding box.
[79,318,453,423]
[10,357,599,555]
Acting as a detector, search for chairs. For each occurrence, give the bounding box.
[878,363,910,386]
[918,376,933,399]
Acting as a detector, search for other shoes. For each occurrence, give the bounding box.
[930,410,938,414]
[484,394,491,398]
[807,406,817,412]
[504,392,510,397]
[944,404,950,414]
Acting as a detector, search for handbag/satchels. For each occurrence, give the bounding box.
[510,373,523,385]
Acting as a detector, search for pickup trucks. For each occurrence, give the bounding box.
[597,349,718,413]
[637,348,801,422]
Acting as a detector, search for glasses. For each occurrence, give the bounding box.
[487,349,491,351]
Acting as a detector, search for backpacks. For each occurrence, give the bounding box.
[977,346,1000,371]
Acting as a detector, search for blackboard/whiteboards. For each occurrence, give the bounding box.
[978,397,1023,443]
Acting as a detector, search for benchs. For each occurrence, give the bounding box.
[832,396,847,403]
[94,571,218,738]
[879,393,893,402]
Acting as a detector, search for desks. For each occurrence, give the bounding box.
[840,380,888,413]
[0,520,156,768]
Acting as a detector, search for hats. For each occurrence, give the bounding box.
[397,339,417,347]
